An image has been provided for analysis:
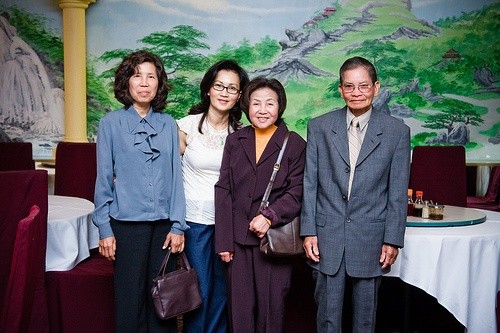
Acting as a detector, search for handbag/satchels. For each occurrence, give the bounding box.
[150,247,202,322]
[257,201,306,257]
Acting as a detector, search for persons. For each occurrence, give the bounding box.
[92,50,188,332]
[215,78,306,333]
[300,56,411,333]
[174,60,253,332]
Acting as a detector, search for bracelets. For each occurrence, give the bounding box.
[266,218,271,227]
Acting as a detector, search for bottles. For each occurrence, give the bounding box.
[413,191,423,217]
[422,200,429,218]
[428,205,444,219]
[406,189,414,216]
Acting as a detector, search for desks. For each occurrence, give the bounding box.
[466,163,500,166]
[35,162,55,195]
[381,205,500,333]
[45,195,100,271]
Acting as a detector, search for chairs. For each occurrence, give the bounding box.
[412,146,500,212]
[0,142,116,333]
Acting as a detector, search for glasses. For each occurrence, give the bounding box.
[341,83,375,93]
[212,84,243,95]
